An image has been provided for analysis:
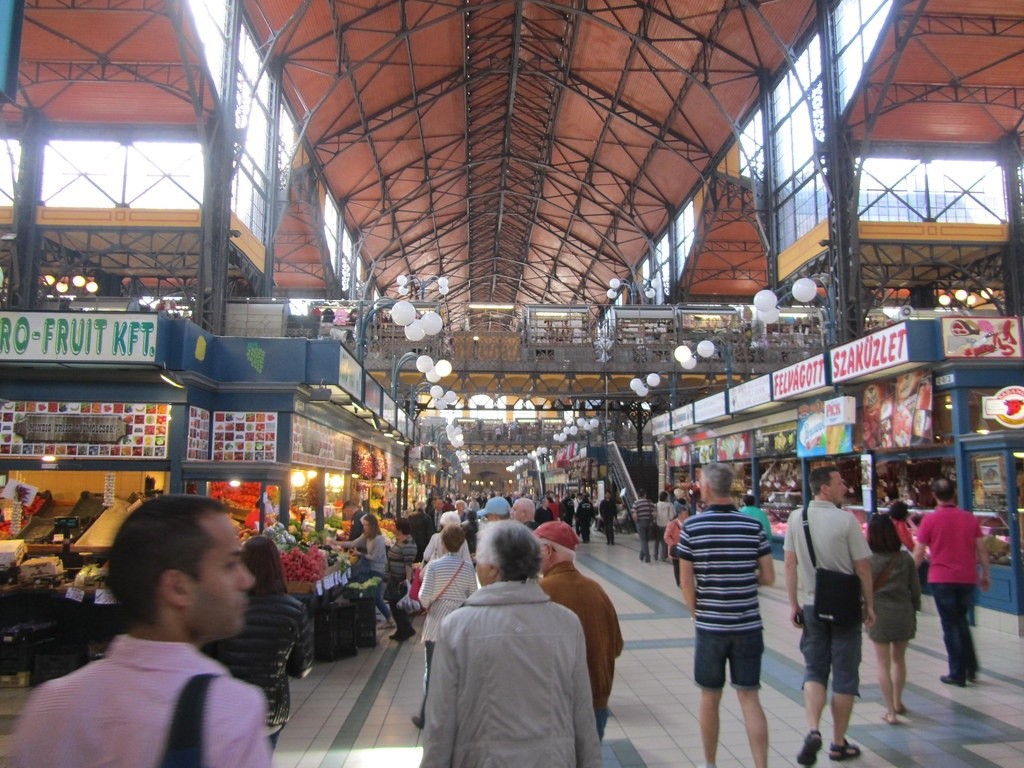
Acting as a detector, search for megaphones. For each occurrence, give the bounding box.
[900,305,914,318]
[329,328,347,342]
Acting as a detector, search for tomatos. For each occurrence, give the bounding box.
[208,481,261,509]
[0,495,45,531]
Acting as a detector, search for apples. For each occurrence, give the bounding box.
[237,529,257,542]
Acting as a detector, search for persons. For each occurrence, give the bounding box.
[330,514,396,629]
[533,521,624,741]
[387,517,417,642]
[673,488,689,511]
[652,491,674,561]
[215,536,314,749]
[630,488,656,563]
[341,501,366,541]
[599,490,618,545]
[888,501,914,552]
[418,520,602,768]
[407,492,596,568]
[411,524,478,729]
[613,503,628,520]
[783,466,876,765]
[738,495,772,541]
[664,507,689,587]
[675,462,776,768]
[864,514,922,724]
[4,495,274,768]
[912,479,989,687]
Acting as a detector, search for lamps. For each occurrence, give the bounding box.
[351,275,471,475]
[309,378,332,403]
[504,276,836,473]
[156,364,184,388]
[43,271,97,293]
[938,287,993,306]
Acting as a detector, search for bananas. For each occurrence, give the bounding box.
[370,486,384,510]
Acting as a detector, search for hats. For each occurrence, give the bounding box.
[533,520,579,551]
[440,510,461,528]
[475,496,511,516]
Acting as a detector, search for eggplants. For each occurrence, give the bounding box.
[300,541,339,566]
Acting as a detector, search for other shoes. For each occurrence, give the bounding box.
[941,674,965,687]
[965,665,977,680]
[412,716,421,728]
[388,627,416,641]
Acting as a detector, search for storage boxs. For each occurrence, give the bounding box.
[0,490,112,578]
[0,626,60,688]
[314,594,378,660]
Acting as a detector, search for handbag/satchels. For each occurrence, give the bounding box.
[647,522,657,541]
[383,574,407,603]
[395,579,421,615]
[813,568,863,628]
[409,567,422,602]
[670,545,677,557]
[351,556,372,580]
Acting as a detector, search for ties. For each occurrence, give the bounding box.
[435,512,440,530]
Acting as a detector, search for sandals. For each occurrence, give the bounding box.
[378,621,395,629]
[829,739,860,761]
[797,731,822,765]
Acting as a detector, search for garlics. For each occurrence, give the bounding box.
[9,501,23,534]
[101,474,115,506]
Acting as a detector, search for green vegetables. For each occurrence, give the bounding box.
[288,515,342,548]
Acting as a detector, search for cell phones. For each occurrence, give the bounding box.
[796,613,804,624]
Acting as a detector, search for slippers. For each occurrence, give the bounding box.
[879,710,898,726]
[894,704,905,714]
